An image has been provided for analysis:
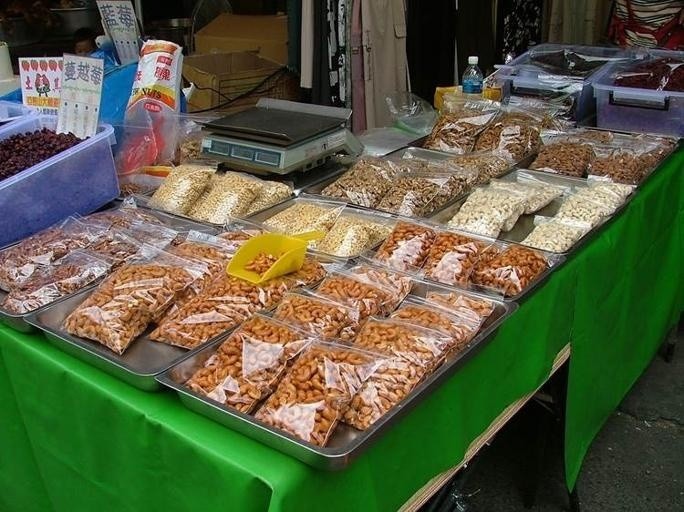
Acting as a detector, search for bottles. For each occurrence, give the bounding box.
[462,56,483,98]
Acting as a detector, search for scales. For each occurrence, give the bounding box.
[192,97,365,189]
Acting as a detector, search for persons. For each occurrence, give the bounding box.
[71,27,94,56]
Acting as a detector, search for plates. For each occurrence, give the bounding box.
[23,230,351,395]
[0,202,224,335]
[418,167,638,261]
[133,188,296,236]
[155,260,520,476]
[355,220,565,304]
[113,173,166,202]
[513,125,680,190]
[229,195,414,267]
[298,145,517,224]
[406,133,541,170]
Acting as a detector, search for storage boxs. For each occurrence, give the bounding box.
[0,50,187,157]
[497,44,625,120]
[0,114,120,250]
[182,51,300,113]
[194,14,288,65]
[591,49,684,139]
[0,100,38,130]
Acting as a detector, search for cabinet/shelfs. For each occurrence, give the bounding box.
[0,145,684,512]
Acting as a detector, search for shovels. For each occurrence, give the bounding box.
[225,229,326,285]
